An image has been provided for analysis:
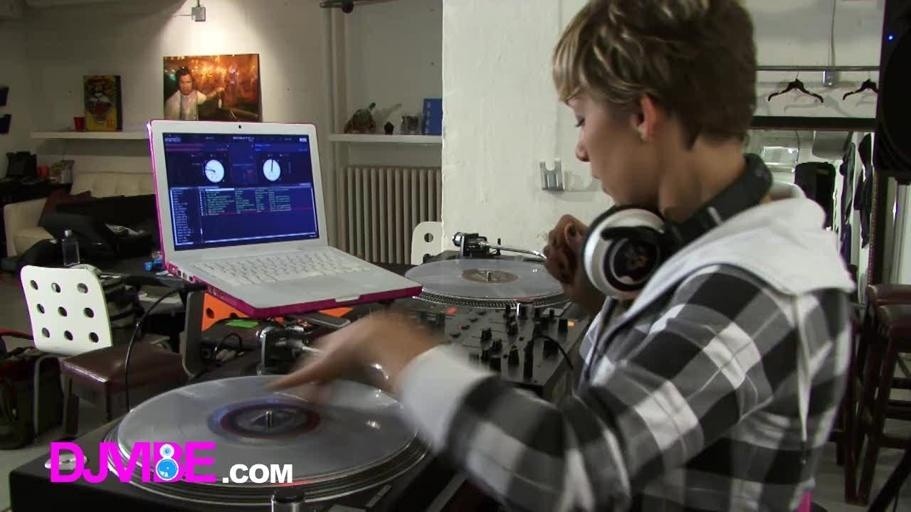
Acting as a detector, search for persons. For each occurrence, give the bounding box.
[264,0,859,510]
[164,68,226,121]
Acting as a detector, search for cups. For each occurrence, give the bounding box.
[73,115,86,131]
[37,165,50,176]
[400,114,422,136]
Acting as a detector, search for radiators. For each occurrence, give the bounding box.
[335,163,440,268]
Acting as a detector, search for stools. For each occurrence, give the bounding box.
[57,342,188,438]
[842,282,910,507]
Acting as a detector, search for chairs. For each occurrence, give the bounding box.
[18,264,172,441]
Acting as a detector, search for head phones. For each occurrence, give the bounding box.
[580,153,771,310]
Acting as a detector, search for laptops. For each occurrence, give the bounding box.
[146,118,423,318]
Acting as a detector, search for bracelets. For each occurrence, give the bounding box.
[215,88,219,95]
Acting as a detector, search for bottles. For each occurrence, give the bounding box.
[344,101,376,133]
[61,229,80,268]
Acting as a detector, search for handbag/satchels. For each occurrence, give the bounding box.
[1,328,65,451]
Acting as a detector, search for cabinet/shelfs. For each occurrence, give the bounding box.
[766,62,880,105]
